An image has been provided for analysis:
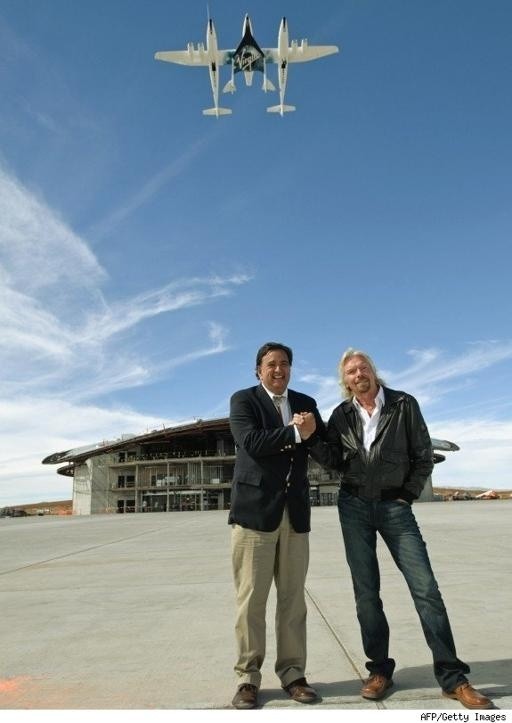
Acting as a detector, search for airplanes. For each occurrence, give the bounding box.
[150,10,343,125]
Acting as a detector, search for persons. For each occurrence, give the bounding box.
[229,343,327,709]
[287,347,493,708]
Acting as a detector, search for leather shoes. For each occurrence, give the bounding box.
[233,683,258,709]
[361,674,393,700]
[284,678,316,703]
[442,680,495,708]
